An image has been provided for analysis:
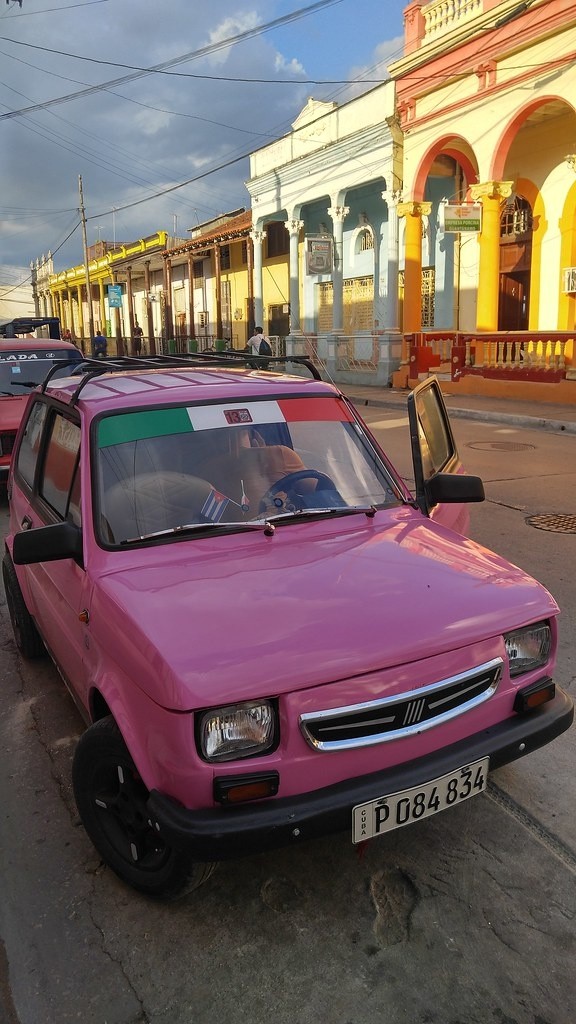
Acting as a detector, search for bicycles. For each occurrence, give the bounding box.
[201,334,238,360]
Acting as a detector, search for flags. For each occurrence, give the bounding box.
[201,490,229,522]
[240,493,250,515]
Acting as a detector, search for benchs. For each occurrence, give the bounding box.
[99,427,265,491]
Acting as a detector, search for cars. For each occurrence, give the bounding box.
[0,337,89,503]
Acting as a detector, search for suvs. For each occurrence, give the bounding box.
[1,345,574,903]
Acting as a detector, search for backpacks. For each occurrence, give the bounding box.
[252,336,272,356]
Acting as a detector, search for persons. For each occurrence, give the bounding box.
[62,329,71,339]
[244,327,272,370]
[60,331,63,338]
[92,331,107,358]
[194,426,316,523]
[132,322,143,356]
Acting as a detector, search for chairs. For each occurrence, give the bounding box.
[198,445,306,521]
[104,470,217,544]
